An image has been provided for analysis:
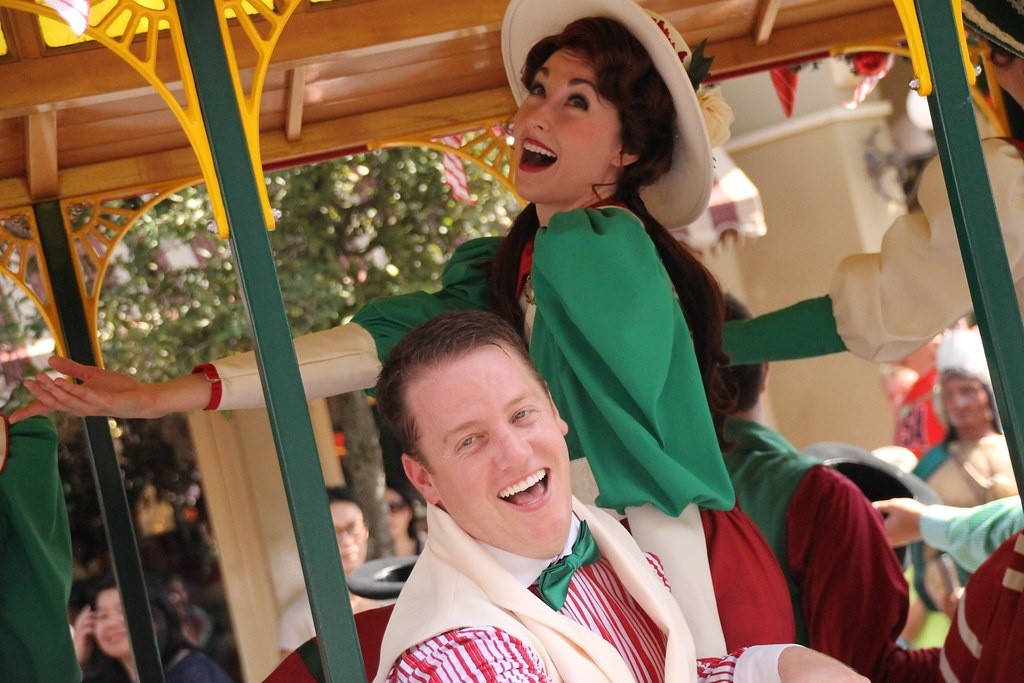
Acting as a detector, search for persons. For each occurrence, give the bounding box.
[712,286,946,682]
[0,409,87,681]
[720,0,1024,367]
[938,527,1024,683]
[73,576,240,683]
[278,485,419,663]
[371,310,876,683]
[880,365,919,406]
[6,0,796,662]
[893,333,949,461]
[874,479,1023,574]
[914,334,1019,620]
[381,484,426,555]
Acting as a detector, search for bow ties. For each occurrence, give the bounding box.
[532,519,604,614]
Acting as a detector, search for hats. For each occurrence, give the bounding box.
[500,1,735,232]
